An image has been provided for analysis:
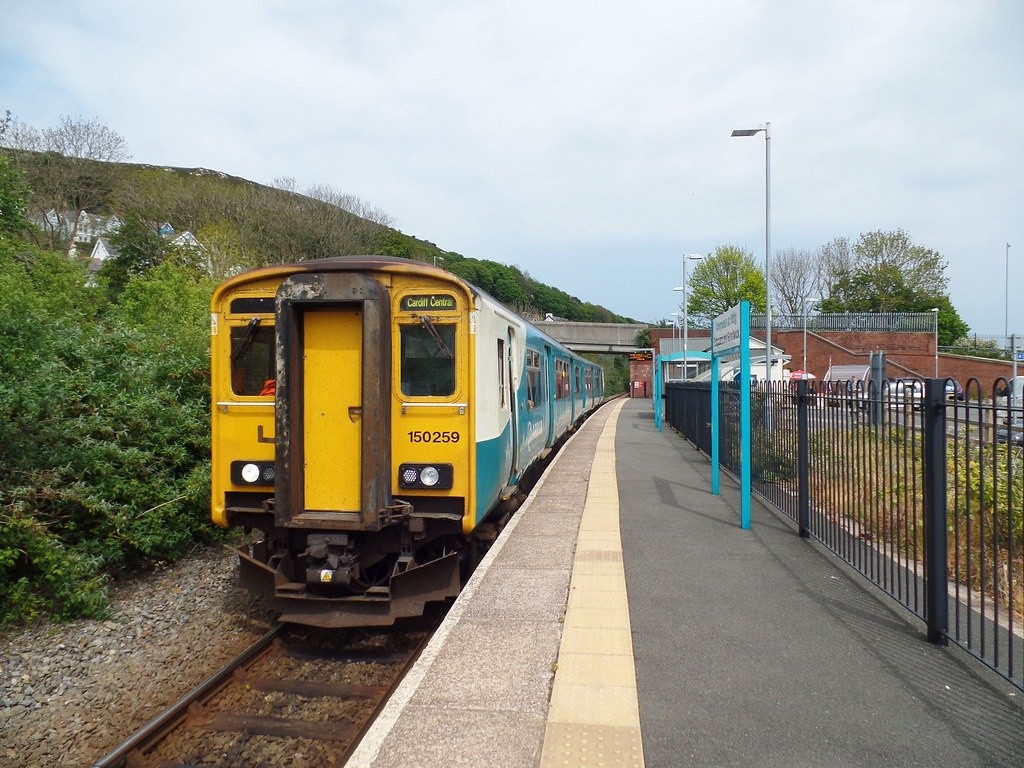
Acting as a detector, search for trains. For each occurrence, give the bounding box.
[208,250,606,630]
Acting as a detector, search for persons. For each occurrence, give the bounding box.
[787,380,797,405]
[826,380,832,404]
[847,376,860,413]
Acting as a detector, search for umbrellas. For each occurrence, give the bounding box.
[784,370,816,380]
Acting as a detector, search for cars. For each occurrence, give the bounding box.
[989,375,1024,447]
[946,378,964,401]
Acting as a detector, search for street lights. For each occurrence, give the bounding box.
[1005,242,1012,356]
[671,313,682,352]
[731,120,773,450]
[930,308,939,379]
[673,286,687,382]
[802,297,819,373]
[682,252,704,381]
